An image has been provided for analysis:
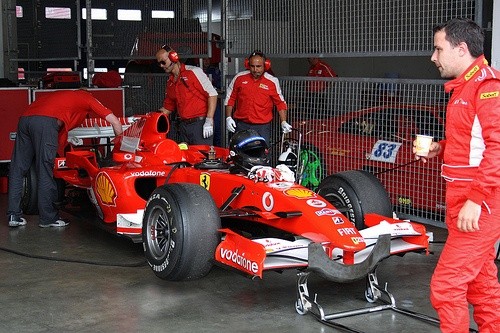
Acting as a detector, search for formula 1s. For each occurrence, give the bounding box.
[51,109,435,283]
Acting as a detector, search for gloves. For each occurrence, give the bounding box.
[281,121,292,133]
[68,134,80,146]
[226,117,236,133]
[203,118,213,138]
[248,166,282,183]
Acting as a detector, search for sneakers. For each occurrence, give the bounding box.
[38,215,71,227]
[7,215,27,226]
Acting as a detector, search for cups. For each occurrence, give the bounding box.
[416,135,434,157]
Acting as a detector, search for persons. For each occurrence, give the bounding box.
[224,51,292,167]
[304,48,336,118]
[412,17,500,333]
[8,90,123,228]
[156,44,218,146]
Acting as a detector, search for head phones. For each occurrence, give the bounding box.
[244,52,271,71]
[161,44,180,63]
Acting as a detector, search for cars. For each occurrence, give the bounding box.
[289,102,445,216]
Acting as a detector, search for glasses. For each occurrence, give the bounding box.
[157,58,169,67]
